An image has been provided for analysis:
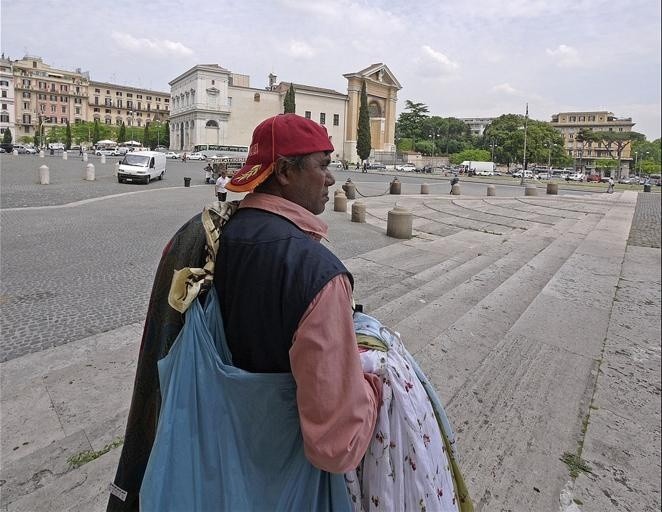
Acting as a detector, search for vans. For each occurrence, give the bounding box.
[115,150,167,186]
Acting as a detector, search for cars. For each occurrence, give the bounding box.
[185,152,206,161]
[512,170,533,179]
[537,168,661,185]
[164,151,181,159]
[94,146,120,156]
[12,144,37,155]
[396,164,416,173]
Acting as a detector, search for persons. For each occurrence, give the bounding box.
[181,152,187,164]
[215,170,230,201]
[606,176,614,193]
[449,175,459,195]
[104,112,381,512]
[79,145,83,157]
[361,162,367,173]
[203,163,214,184]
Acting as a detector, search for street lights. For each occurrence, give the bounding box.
[128,110,137,151]
[39,118,51,150]
[155,119,162,150]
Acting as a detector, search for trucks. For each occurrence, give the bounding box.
[458,160,494,174]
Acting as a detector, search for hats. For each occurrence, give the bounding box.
[224,113,334,192]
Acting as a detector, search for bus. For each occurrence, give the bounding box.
[191,143,251,162]
